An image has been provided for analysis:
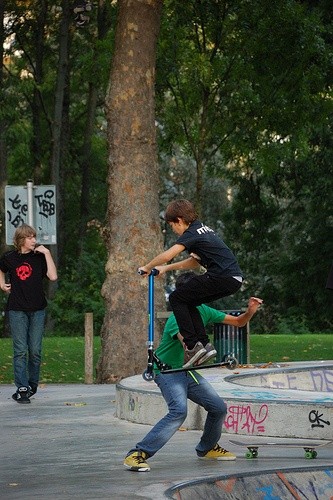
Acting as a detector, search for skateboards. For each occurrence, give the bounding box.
[228,440,333,459]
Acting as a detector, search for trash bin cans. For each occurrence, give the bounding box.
[214,310,250,366]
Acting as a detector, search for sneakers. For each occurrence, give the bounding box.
[198,444,237,460]
[123,451,150,472]
[181,342,218,369]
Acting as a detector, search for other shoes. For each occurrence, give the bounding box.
[11,384,37,404]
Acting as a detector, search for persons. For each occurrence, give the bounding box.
[121,273,264,472]
[0,224,58,404]
[137,198,245,368]
